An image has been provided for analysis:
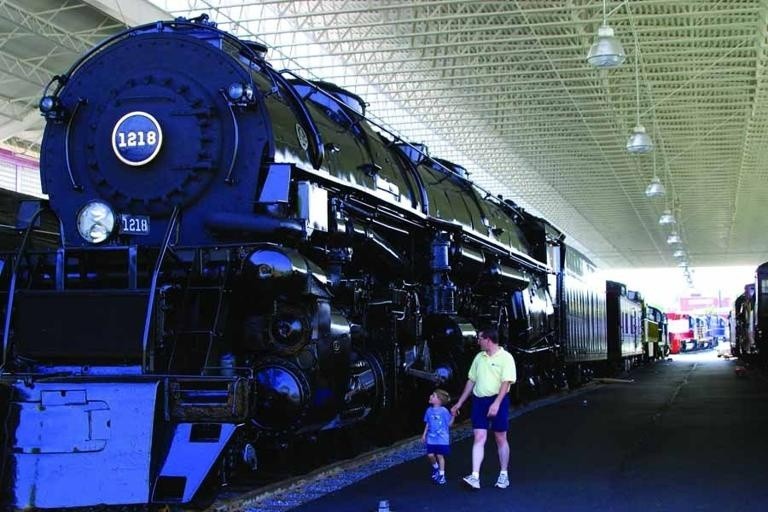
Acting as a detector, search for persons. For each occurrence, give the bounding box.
[421,389,457,485]
[449,327,517,489]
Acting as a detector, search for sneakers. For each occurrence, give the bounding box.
[432,466,446,485]
[463,475,480,489]
[495,476,510,489]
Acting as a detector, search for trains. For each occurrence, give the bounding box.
[0,13,768,512]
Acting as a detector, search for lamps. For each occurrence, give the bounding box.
[584,0,627,70]
[644,118,666,198]
[660,166,693,284]
[626,30,653,156]
